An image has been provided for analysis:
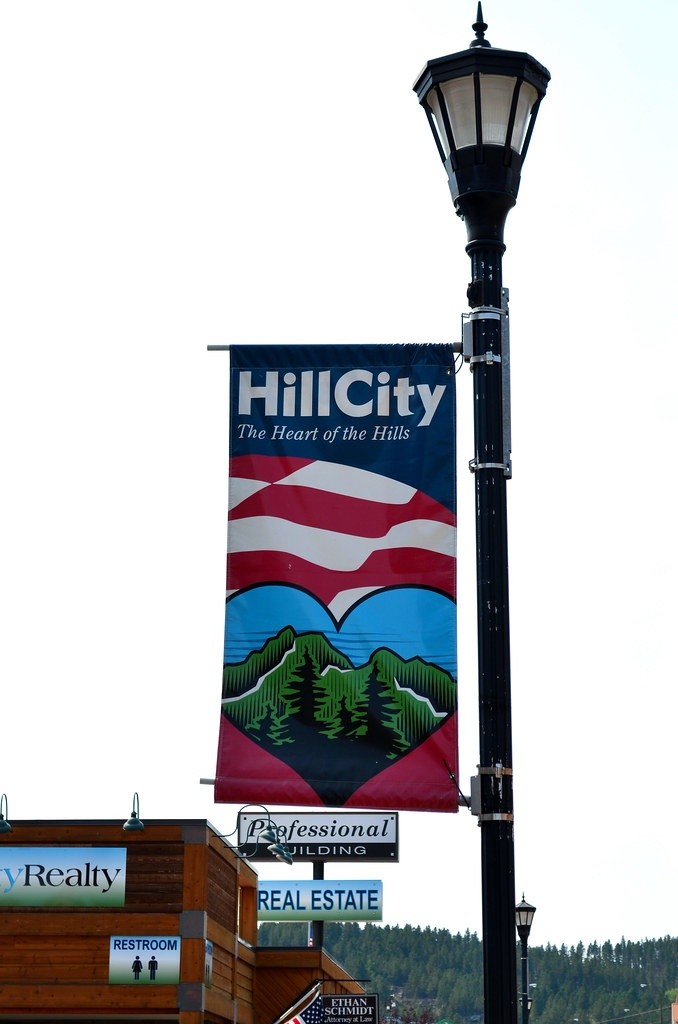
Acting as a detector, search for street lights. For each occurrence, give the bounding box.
[519,998,533,1024]
[515,892,536,1023]
[413,1,554,1024]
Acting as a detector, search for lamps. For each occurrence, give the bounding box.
[0,794,14,833]
[212,804,294,866]
[122,792,145,832]
